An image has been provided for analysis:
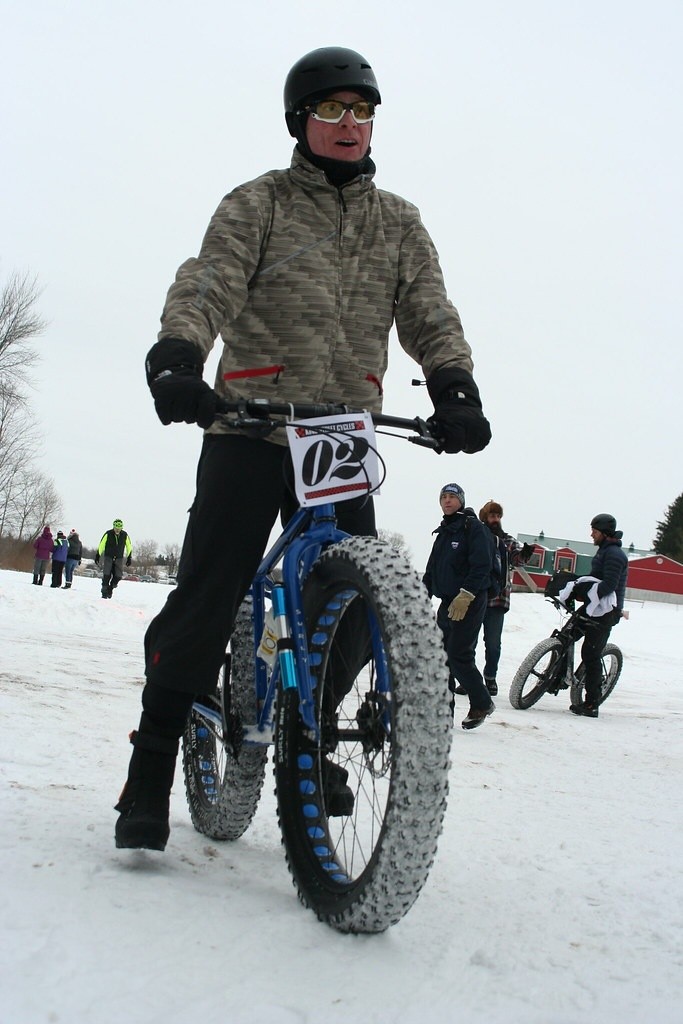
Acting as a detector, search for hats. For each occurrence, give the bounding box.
[439,483,465,507]
[112,518,123,529]
[590,514,616,534]
[43,525,50,532]
[57,530,63,536]
[69,529,76,535]
[479,500,503,522]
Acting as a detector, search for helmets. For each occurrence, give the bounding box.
[284,47,381,137]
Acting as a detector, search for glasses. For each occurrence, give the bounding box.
[296,100,375,123]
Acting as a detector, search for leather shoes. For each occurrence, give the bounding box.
[462,699,496,730]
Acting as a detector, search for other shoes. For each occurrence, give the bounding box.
[102,585,113,599]
[61,582,72,589]
[50,584,61,588]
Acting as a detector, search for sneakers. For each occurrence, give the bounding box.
[454,684,467,695]
[569,694,598,718]
[321,756,354,809]
[114,731,179,851]
[484,676,498,696]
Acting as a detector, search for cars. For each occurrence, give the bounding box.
[82,569,177,585]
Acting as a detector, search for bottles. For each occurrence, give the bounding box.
[255,607,277,666]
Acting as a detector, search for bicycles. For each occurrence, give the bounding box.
[508,591,623,715]
[181,391,454,934]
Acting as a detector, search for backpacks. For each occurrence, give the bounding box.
[488,536,509,599]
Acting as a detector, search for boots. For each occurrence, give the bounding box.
[31,574,44,585]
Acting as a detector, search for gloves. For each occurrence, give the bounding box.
[78,561,81,565]
[447,588,476,621]
[411,367,492,455]
[126,558,131,567]
[95,556,100,565]
[144,339,220,431]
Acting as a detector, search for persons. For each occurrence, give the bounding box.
[541,514,628,718]
[95,520,132,599]
[422,483,496,730]
[113,46,492,851]
[455,502,535,695]
[50,531,68,588]
[61,529,82,589]
[32,526,54,585]
